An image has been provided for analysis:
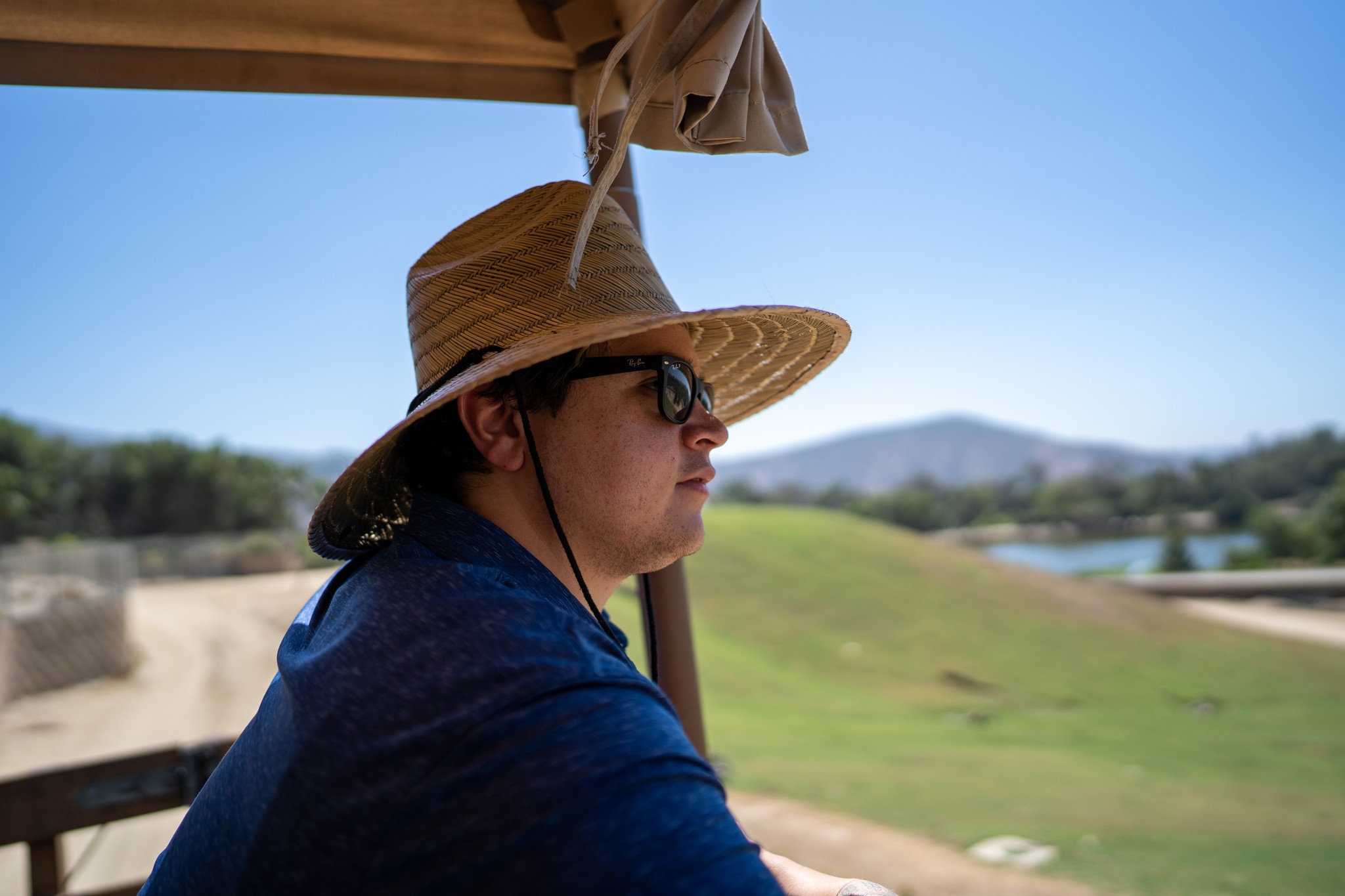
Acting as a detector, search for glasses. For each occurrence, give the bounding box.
[540,353,714,424]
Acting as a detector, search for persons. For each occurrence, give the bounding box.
[132,205,892,896]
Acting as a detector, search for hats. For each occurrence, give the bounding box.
[307,179,853,562]
